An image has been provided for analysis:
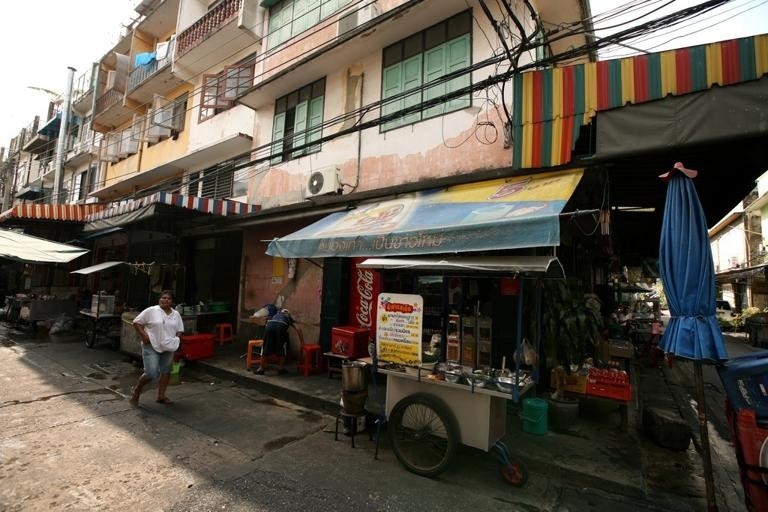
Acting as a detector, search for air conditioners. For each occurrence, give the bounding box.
[303,165,341,199]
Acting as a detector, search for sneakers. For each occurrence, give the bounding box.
[254,370,288,374]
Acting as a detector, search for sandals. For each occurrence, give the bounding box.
[130,387,139,406]
[157,396,174,404]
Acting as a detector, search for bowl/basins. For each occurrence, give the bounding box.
[467,374,492,387]
[403,360,438,377]
[170,363,181,374]
[444,369,462,382]
[494,377,526,393]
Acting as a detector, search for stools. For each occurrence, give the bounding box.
[210,321,322,379]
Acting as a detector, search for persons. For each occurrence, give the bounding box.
[130,289,185,406]
[253,308,304,374]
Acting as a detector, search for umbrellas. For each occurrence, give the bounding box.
[657,162,730,511]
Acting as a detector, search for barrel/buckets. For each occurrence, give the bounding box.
[341,413,368,434]
[341,392,370,413]
[519,397,548,435]
